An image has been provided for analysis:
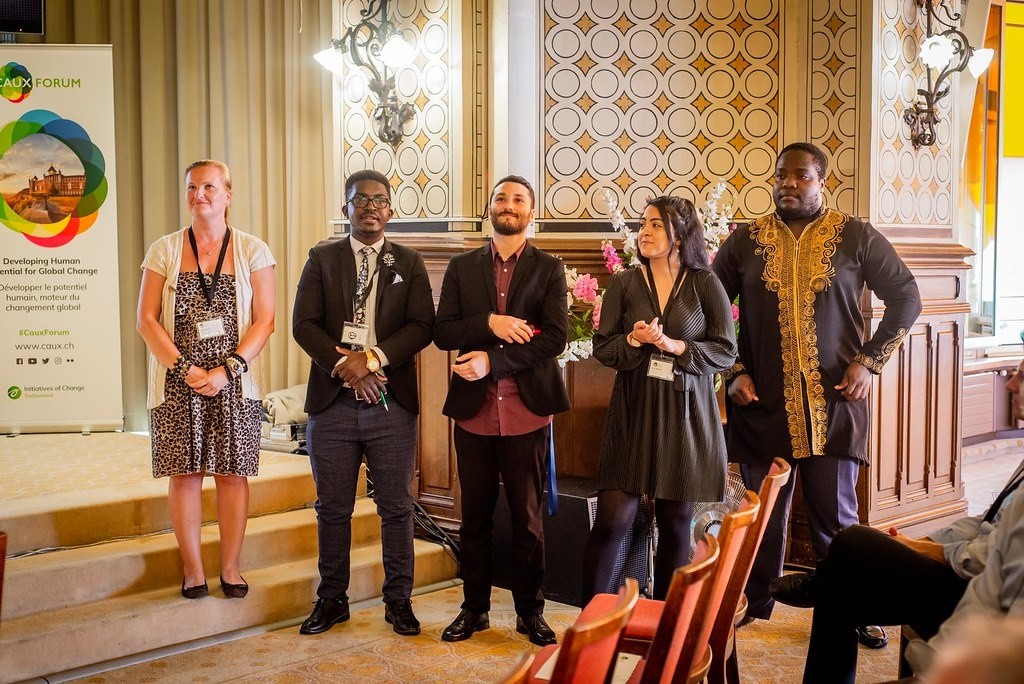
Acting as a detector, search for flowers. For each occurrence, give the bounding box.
[556,174,740,392]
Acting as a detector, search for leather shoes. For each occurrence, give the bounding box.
[516,615,557,646]
[384,600,421,636]
[441,608,489,641]
[859,624,888,648]
[301,596,350,634]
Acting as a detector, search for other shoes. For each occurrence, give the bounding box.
[219,572,249,599]
[768,572,815,608]
[181,574,209,599]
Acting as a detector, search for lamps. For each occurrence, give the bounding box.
[901,0,994,146]
[312,0,417,147]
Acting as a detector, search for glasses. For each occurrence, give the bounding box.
[346,193,392,210]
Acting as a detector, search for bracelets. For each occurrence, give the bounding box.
[232,353,247,372]
[171,355,192,381]
[222,362,234,383]
[488,313,493,327]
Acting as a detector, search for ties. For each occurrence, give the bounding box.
[351,248,375,352]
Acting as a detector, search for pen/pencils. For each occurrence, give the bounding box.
[379,390,389,411]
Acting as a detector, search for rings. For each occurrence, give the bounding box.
[467,374,471,378]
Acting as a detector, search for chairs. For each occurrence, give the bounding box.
[501,456,791,684]
[899,623,938,679]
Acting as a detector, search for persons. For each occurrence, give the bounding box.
[769,331,1024,684]
[712,142,923,649]
[919,608,1024,684]
[135,159,276,600]
[434,174,568,647]
[292,169,435,634]
[582,196,738,613]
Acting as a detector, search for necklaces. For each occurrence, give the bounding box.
[196,239,222,256]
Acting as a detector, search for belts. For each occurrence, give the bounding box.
[340,384,390,401]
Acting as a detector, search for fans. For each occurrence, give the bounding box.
[690,471,745,552]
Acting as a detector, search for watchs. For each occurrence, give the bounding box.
[227,358,242,375]
[366,350,379,372]
[629,334,644,347]
